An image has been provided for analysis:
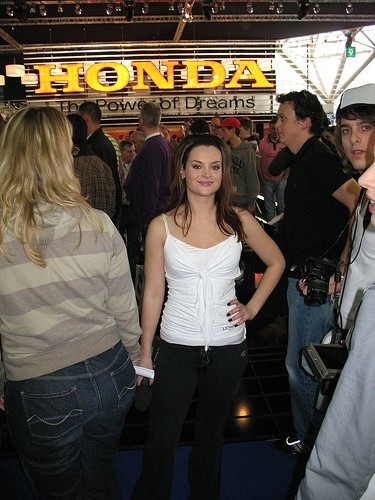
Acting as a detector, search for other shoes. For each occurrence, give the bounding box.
[276,437,312,455]
[267,422,296,440]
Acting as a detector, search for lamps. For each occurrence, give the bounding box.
[5,56,25,77]
[0,74,5,86]
[21,66,39,85]
[5,0,353,22]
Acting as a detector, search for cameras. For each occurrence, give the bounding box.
[303,257,338,306]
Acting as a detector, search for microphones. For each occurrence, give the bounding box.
[134,347,154,411]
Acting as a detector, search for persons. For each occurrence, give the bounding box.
[132,133,285,500]
[60,99,338,311]
[0,105,142,500]
[323,82,375,346]
[296,161,375,500]
[270,90,353,456]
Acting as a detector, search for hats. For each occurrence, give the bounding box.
[215,117,241,129]
[336,83,375,119]
